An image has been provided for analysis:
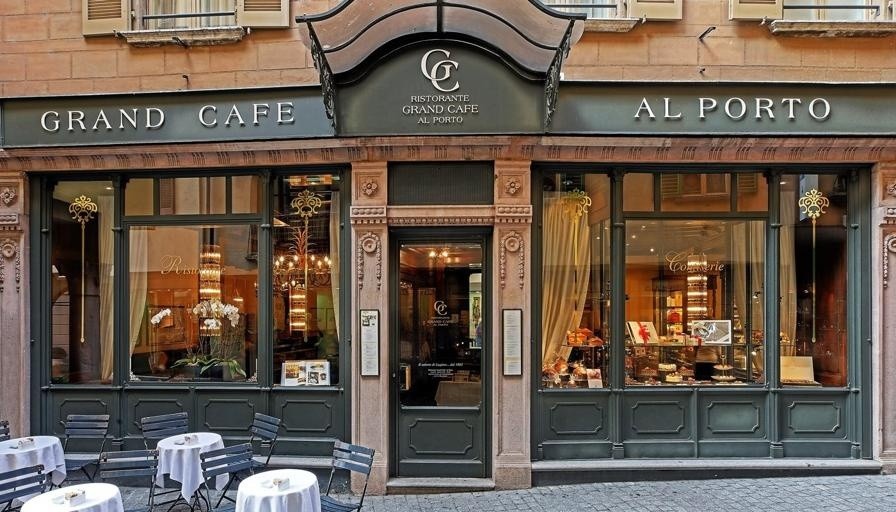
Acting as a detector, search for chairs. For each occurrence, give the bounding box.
[0,464,45,512]
[320,441,375,512]
[0,420,10,442]
[141,411,198,511]
[213,412,281,509]
[200,442,254,512]
[49,414,110,492]
[98,450,160,512]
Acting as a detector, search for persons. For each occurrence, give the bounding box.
[694,346,722,380]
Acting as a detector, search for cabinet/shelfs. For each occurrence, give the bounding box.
[290,284,317,339]
[625,343,764,387]
[199,244,226,302]
[666,274,713,335]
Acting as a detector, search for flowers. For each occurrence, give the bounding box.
[150,298,247,377]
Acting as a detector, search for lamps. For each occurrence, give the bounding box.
[273,230,331,292]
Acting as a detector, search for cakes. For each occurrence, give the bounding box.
[658,362,676,372]
[665,371,683,383]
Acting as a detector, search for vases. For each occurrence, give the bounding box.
[180,364,229,381]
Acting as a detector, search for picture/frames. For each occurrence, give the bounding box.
[691,319,733,345]
[502,308,523,377]
[360,310,380,377]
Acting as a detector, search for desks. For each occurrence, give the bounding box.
[151,432,230,511]
[0,435,67,503]
[434,381,481,406]
[235,469,321,512]
[19,482,125,512]
